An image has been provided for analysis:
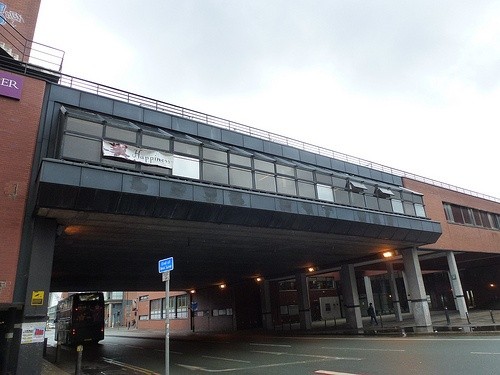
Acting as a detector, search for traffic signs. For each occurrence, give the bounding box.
[158,256,174,273]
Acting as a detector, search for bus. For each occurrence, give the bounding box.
[55,293,105,348]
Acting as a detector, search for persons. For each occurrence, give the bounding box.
[368,303,378,326]
